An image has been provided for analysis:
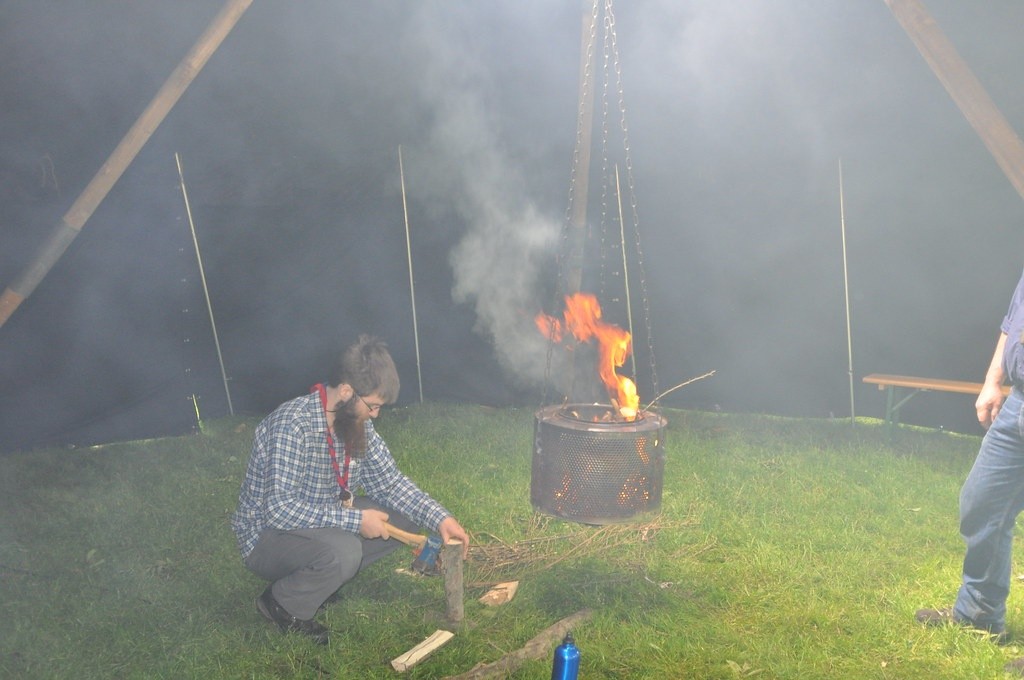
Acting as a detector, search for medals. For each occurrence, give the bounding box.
[340,490,351,501]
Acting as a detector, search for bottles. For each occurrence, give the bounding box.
[551,631,580,680]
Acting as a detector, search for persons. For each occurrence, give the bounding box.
[914,272,1024,675]
[231,345,470,645]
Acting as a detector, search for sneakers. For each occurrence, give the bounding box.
[257,580,331,646]
[1004,658,1024,676]
[915,609,1007,643]
[321,592,345,610]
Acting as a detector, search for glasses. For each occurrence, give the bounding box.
[343,383,384,418]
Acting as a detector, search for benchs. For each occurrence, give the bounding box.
[862,372,1013,426]
